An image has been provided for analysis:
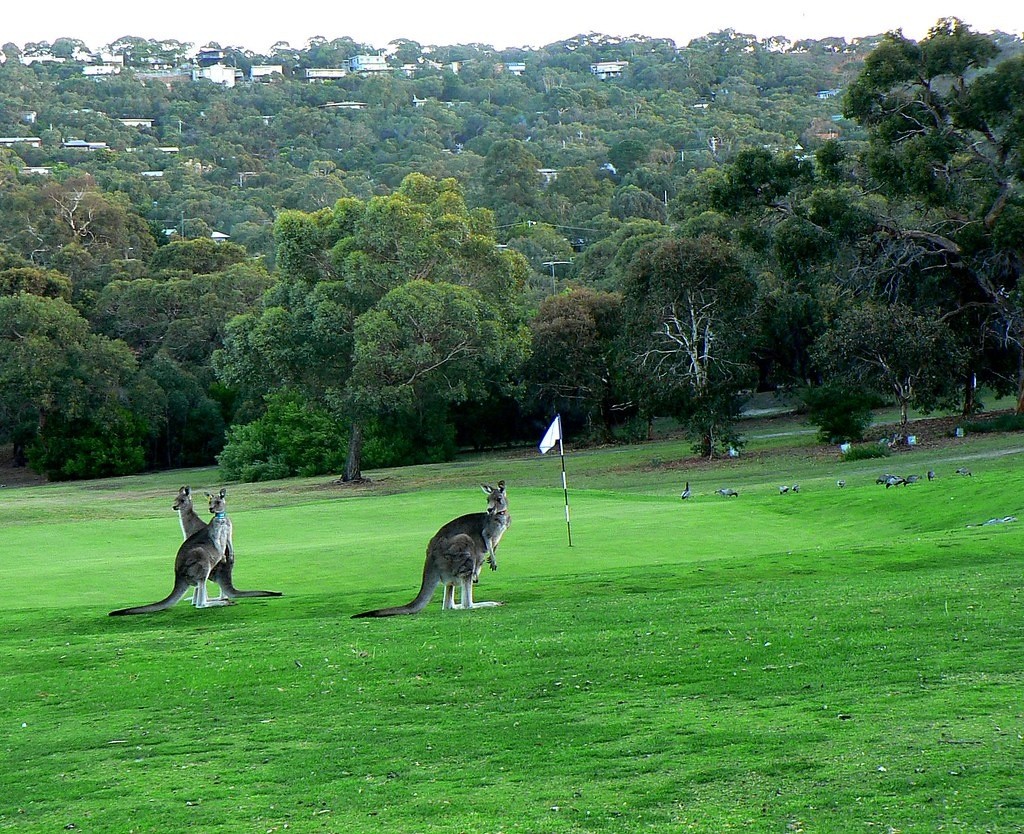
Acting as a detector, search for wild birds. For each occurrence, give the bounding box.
[715,488,738,498]
[967,516,1019,528]
[876,471,934,489]
[681,482,690,499]
[779,484,800,494]
[955,468,972,476]
[837,480,845,488]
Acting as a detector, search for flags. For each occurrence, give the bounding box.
[539,416,560,454]
